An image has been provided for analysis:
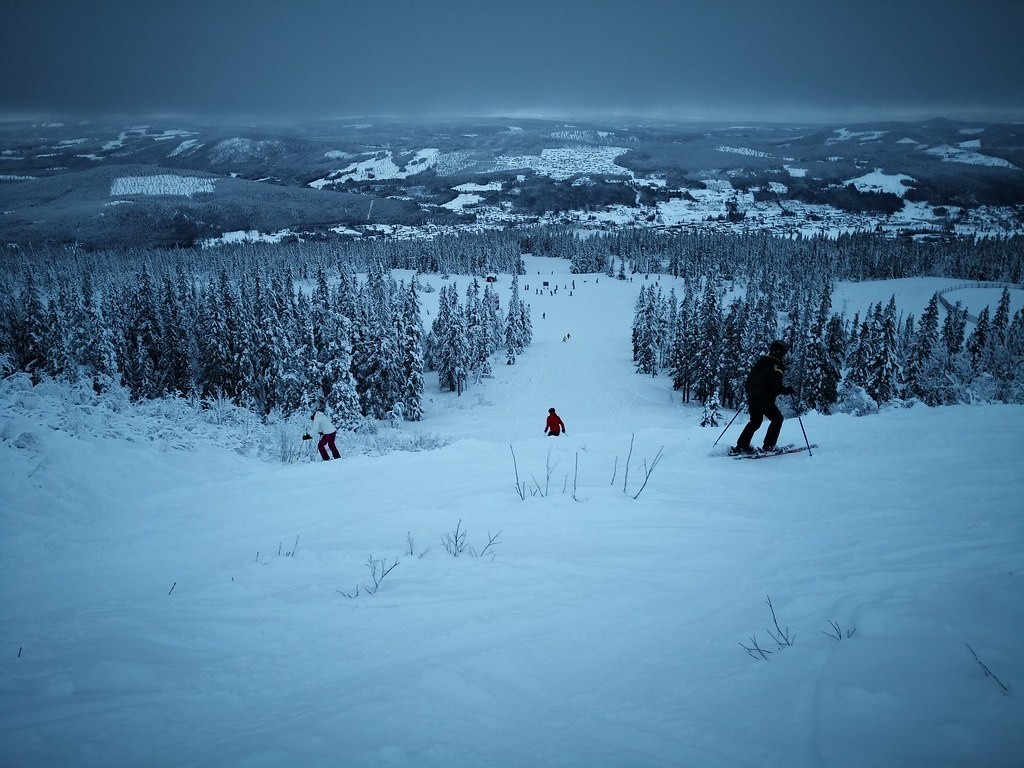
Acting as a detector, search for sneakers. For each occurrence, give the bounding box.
[763,444,781,452]
[737,445,755,453]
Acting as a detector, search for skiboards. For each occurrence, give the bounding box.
[706,443,819,460]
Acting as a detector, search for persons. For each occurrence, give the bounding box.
[302,409,342,460]
[544,407,566,437]
[736,341,796,453]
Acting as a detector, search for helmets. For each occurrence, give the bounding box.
[771,340,790,357]
[306,410,314,420]
